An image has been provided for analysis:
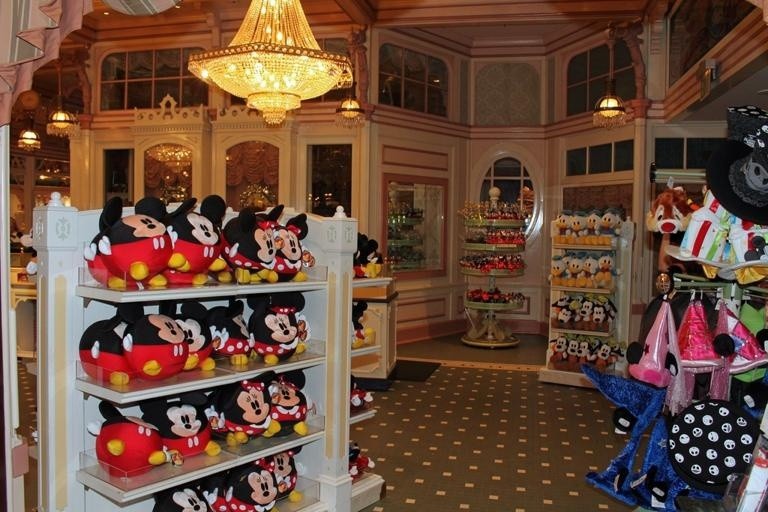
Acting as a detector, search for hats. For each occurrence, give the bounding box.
[581,297,767,512]
[646,107,767,286]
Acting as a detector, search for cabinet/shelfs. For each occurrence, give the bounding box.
[460,186,527,349]
[538,216,634,389]
[32,192,387,512]
[388,202,425,269]
[351,276,398,379]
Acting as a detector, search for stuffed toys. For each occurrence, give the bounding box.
[348,439,377,476]
[86,369,312,477]
[80,292,309,386]
[547,206,628,374]
[352,300,377,347]
[84,196,313,290]
[77,196,314,510]
[14,226,38,277]
[151,446,303,512]
[644,189,693,235]
[353,233,382,281]
[350,373,373,410]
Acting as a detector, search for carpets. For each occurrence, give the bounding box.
[388,360,441,381]
[355,377,393,391]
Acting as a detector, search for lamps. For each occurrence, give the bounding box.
[18,116,41,151]
[331,58,353,88]
[336,89,365,119]
[46,64,80,137]
[591,40,627,129]
[188,0,348,124]
[146,143,192,174]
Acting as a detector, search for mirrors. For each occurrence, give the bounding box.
[301,135,359,218]
[382,173,448,280]
